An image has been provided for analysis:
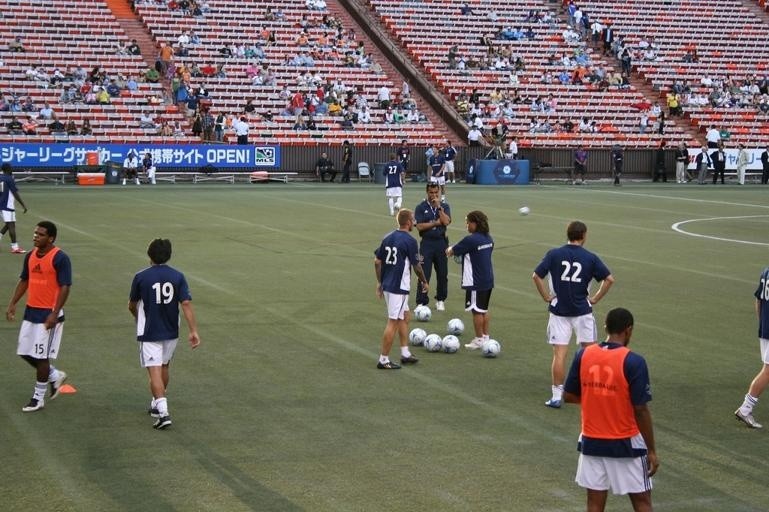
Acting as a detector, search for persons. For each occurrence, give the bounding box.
[562,307,659,511]
[640,112,730,149]
[448,3,632,158]
[382,152,406,217]
[734,265,769,429]
[639,36,768,117]
[315,153,336,183]
[445,209,496,351]
[373,206,429,369]
[412,180,452,312]
[611,143,627,186]
[573,145,587,185]
[340,140,352,184]
[4,221,73,412]
[0,36,419,146]
[531,221,615,408]
[128,237,202,430]
[167,0,357,48]
[122,152,156,186]
[396,139,457,183]
[0,161,28,254]
[654,141,768,185]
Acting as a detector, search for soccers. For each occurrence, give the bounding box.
[448,318,464,335]
[519,207,531,216]
[424,334,443,353]
[443,335,459,354]
[416,306,431,322]
[410,328,427,345]
[482,340,501,357]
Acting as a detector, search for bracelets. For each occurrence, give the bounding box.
[54,310,58,314]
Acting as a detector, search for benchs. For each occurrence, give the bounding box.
[1,170,300,184]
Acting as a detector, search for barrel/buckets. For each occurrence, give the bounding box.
[87,152,99,166]
[374,162,388,186]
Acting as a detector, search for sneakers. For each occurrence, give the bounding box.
[734,408,761,428]
[435,301,445,310]
[377,361,401,369]
[400,354,418,363]
[148,400,172,430]
[22,371,68,412]
[544,400,561,408]
[11,247,26,253]
[464,339,489,350]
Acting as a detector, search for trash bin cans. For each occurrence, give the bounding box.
[106,160,123,184]
[375,163,387,184]
